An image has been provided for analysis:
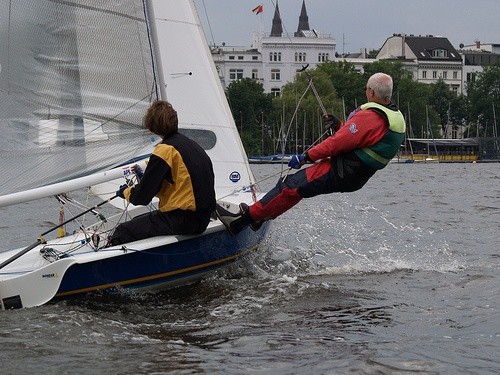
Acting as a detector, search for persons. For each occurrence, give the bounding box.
[112,100,215,246]
[215,72,406,237]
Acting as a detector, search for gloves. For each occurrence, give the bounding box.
[323,113,342,132]
[288,151,313,170]
[116,184,128,199]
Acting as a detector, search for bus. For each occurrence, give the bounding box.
[402,138,480,160]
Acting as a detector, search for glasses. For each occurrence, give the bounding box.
[363,86,372,91]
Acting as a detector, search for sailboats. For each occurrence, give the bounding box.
[1,0,270,313]
[249,91,416,164]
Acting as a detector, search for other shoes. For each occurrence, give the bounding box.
[215,203,249,239]
[238,202,264,232]
